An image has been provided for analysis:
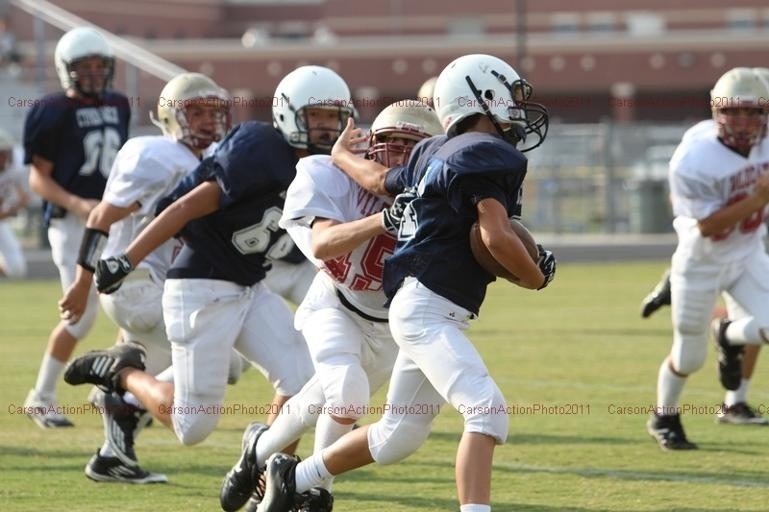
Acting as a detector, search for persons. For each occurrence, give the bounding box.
[220,100,445,512]
[640,268,769,425]
[647,67,769,451]
[58,72,231,486]
[0,130,33,280]
[61,65,362,512]
[22,27,132,428]
[252,54,551,510]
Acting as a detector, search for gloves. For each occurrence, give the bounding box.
[94,253,131,297]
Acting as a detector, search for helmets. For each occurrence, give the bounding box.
[369,97,444,159]
[712,64,769,110]
[271,64,358,148]
[53,27,117,98]
[431,54,550,149]
[152,72,233,143]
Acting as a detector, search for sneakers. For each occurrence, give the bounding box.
[300,484,334,512]
[64,340,149,395]
[716,401,768,424]
[84,449,169,485]
[645,410,696,452]
[22,386,75,432]
[641,269,671,318]
[711,317,748,390]
[87,385,150,468]
[257,452,298,512]
[219,420,270,512]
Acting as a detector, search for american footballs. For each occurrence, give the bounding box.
[470,219,541,289]
